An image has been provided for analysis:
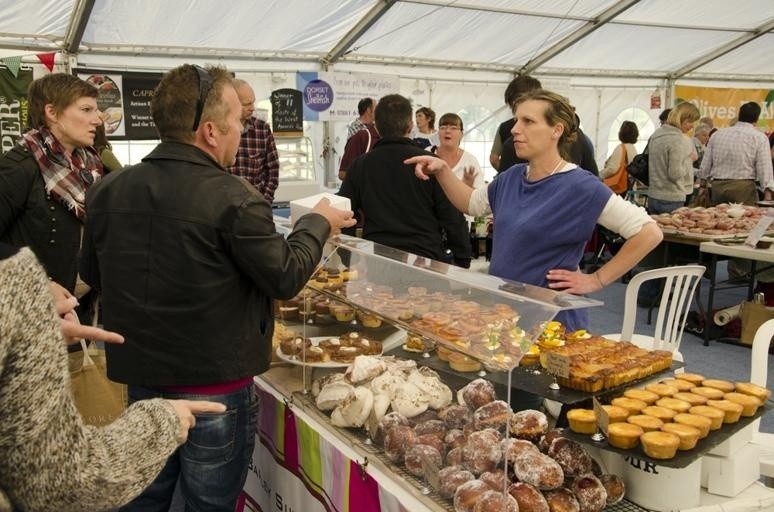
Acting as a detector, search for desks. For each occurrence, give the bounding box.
[636,196,773,341]
[696,233,774,346]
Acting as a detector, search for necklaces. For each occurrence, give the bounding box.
[525,157,564,181]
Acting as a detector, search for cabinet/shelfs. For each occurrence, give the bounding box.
[251,214,774,510]
[268,132,322,218]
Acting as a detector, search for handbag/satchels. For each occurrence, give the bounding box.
[625,153,652,186]
[601,143,629,194]
[65,310,129,429]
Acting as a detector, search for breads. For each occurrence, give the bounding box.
[649,203,774,235]
[274,265,596,373]
[309,355,624,511]
[540,335,673,392]
[565,372,770,461]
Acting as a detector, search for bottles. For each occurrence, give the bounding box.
[484,222,493,261]
[468,221,479,260]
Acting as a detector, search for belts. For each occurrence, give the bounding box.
[714,178,733,181]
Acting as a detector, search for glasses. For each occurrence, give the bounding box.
[191,65,214,135]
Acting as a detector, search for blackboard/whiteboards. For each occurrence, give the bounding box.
[272,89,303,137]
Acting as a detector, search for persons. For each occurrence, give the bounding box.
[76,64,359,512]
[1,233,230,512]
[334,93,472,294]
[334,75,572,234]
[74,117,124,179]
[599,97,774,334]
[223,76,281,205]
[402,87,665,335]
[0,73,106,355]
[575,113,594,154]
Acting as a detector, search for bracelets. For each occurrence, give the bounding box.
[594,270,607,289]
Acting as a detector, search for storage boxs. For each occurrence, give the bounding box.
[740,300,774,349]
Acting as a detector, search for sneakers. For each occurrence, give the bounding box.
[622,273,631,284]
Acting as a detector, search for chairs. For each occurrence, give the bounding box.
[729,317,774,477]
[593,189,647,260]
[585,263,710,379]
